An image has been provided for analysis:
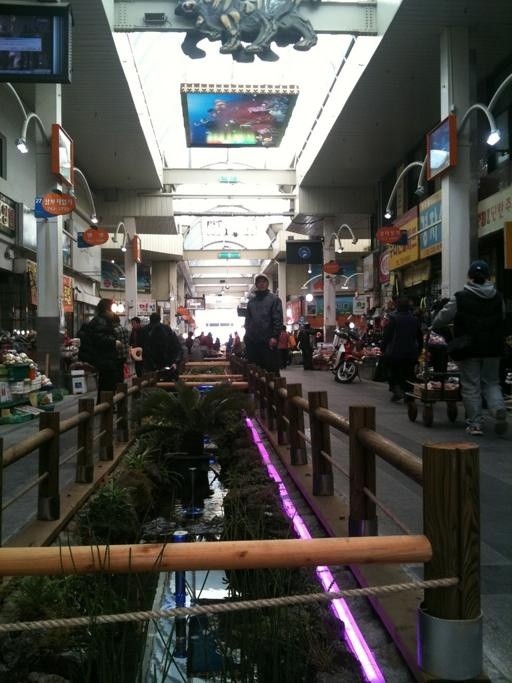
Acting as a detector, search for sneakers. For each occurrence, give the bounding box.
[493,406,509,435]
[391,392,416,404]
[464,425,486,437]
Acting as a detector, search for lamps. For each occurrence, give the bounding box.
[300,272,363,290]
[328,223,358,254]
[111,222,133,252]
[382,71,512,220]
[0,81,99,226]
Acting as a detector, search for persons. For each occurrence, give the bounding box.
[226,333,242,359]
[427,261,510,433]
[375,295,425,401]
[186,330,222,360]
[243,273,297,376]
[76,297,182,422]
[298,322,324,371]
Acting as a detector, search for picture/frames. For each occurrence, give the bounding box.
[51,122,75,189]
[426,115,457,182]
[133,235,141,264]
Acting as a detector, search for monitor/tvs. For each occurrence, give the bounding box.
[0,0,75,85]
[286,240,323,265]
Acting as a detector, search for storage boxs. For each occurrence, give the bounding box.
[0,363,54,418]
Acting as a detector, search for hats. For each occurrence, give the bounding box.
[468,260,490,280]
[255,273,269,282]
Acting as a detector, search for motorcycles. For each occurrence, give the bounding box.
[330,319,363,384]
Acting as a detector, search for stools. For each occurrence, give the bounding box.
[72,377,88,394]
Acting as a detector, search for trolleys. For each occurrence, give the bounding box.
[404,330,466,423]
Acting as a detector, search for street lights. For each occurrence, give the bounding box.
[111,301,124,323]
[283,306,295,334]
